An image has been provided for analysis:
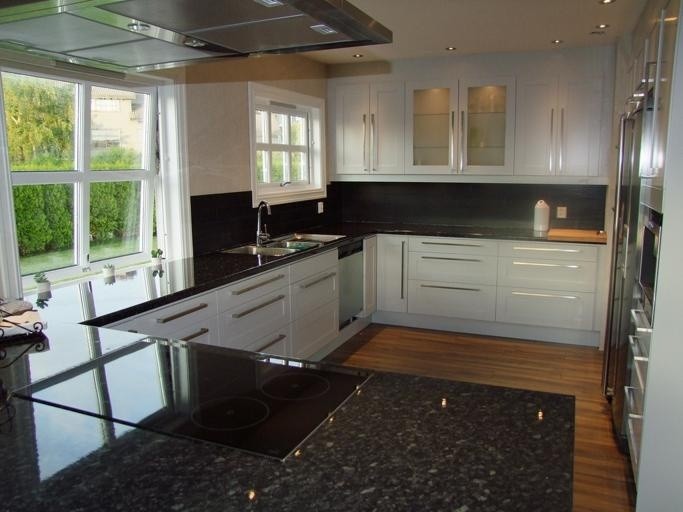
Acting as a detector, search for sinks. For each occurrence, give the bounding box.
[220,246,300,257]
[259,241,322,249]
[271,233,346,241]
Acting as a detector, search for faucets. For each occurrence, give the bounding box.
[256,200,272,245]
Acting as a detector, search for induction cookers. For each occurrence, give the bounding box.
[11,337,374,461]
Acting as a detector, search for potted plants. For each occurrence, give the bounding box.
[36,291,52,309]
[34,271,50,293]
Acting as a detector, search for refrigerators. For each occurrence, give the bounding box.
[598,88,653,445]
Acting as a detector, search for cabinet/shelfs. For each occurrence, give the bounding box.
[404,52,516,184]
[618,300,683,512]
[101,250,341,359]
[326,57,405,182]
[514,44,614,185]
[377,234,610,351]
[615,0,683,213]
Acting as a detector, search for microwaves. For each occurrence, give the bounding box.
[637,215,663,309]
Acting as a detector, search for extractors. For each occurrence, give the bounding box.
[0,0,392,74]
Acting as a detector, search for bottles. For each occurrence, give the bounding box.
[533,200,550,232]
